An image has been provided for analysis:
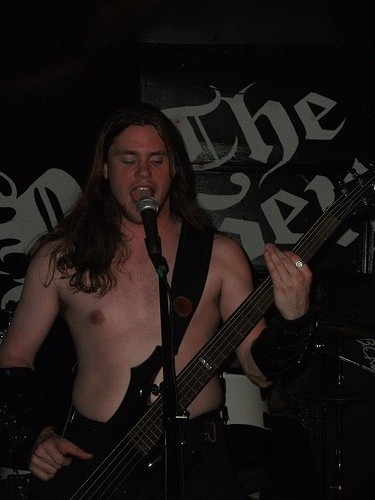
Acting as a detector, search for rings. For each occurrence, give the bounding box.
[294,260,306,270]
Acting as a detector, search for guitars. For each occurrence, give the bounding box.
[61,161,375,500]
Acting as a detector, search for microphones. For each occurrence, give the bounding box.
[137,196,162,258]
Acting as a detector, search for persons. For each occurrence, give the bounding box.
[0,103,313,500]
